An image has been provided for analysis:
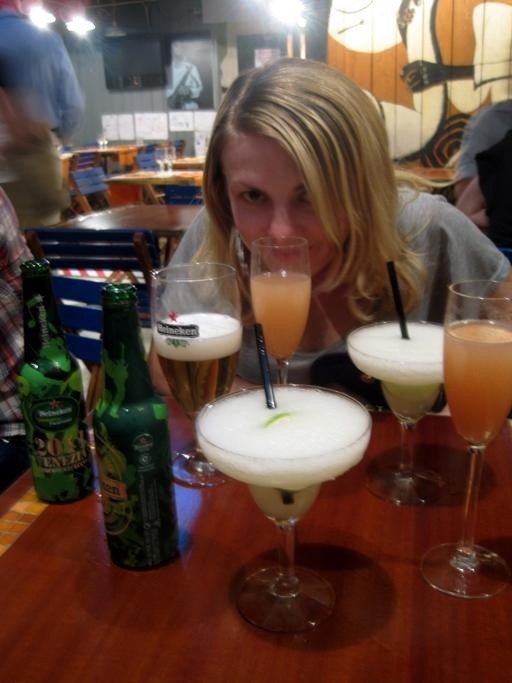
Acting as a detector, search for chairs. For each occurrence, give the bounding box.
[164,183,202,203]
[70,166,111,213]
[73,150,99,168]
[136,139,163,202]
[24,225,164,365]
[163,137,186,153]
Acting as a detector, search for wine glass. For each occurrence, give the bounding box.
[193,382,374,635]
[96,132,109,150]
[346,320,443,504]
[154,146,176,176]
[150,262,243,486]
[249,235,312,383]
[421,279,511,599]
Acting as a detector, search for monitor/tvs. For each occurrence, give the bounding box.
[102,31,167,90]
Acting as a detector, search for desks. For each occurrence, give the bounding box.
[51,203,204,265]
[76,143,140,173]
[101,170,204,204]
[2,393,510,681]
[163,153,206,167]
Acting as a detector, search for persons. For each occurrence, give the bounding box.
[1,0,83,238]
[2,178,50,496]
[446,98,512,204]
[456,125,512,251]
[162,47,204,110]
[143,55,511,408]
[394,0,511,103]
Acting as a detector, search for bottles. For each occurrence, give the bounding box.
[12,256,94,503]
[92,282,179,570]
